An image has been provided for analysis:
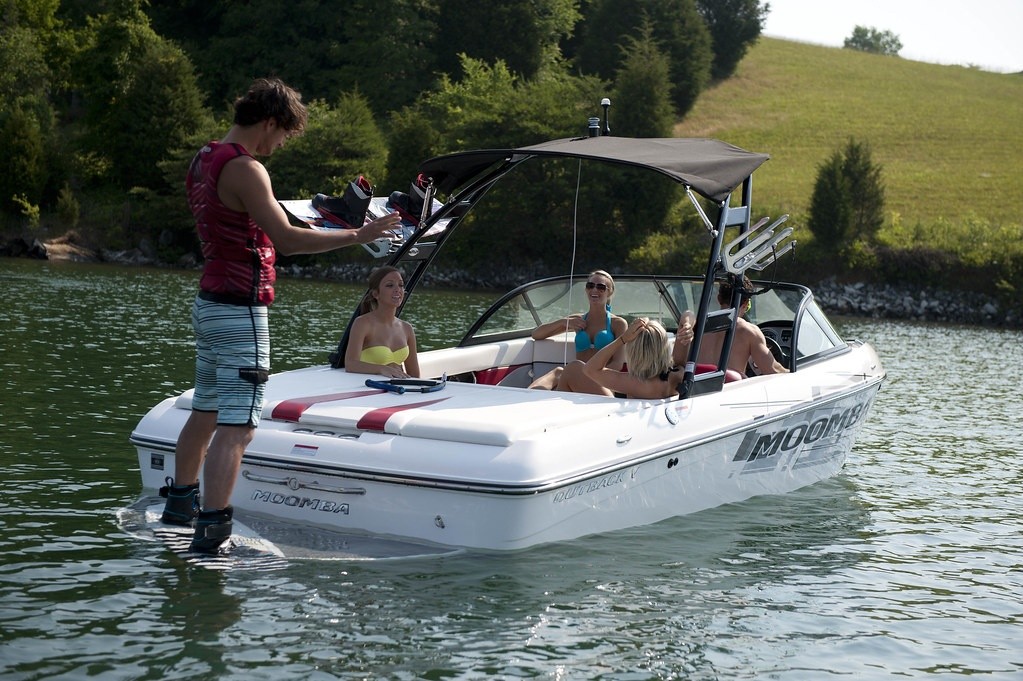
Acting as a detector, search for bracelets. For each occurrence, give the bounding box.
[619,335,626,345]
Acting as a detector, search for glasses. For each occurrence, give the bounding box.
[585,282,611,293]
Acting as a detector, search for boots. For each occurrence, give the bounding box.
[189,506,275,559]
[390,173,438,225]
[311,176,374,229]
[158,475,203,528]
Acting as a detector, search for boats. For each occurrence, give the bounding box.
[129,99,887,552]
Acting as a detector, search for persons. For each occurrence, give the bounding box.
[345,267,421,379]
[527,269,629,397]
[158,77,402,560]
[555,310,697,400]
[696,273,791,377]
